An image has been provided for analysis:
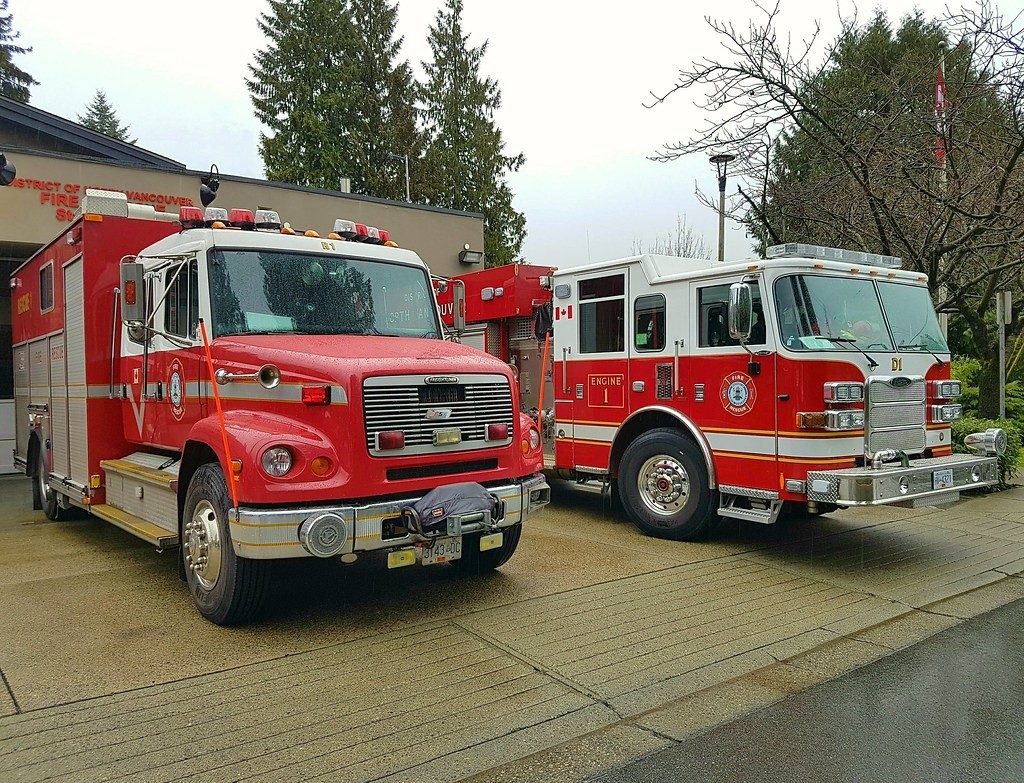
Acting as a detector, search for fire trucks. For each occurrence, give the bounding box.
[9,187,551,628]
[429,243,1008,541]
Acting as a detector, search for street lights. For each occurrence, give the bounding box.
[711,154,736,262]
